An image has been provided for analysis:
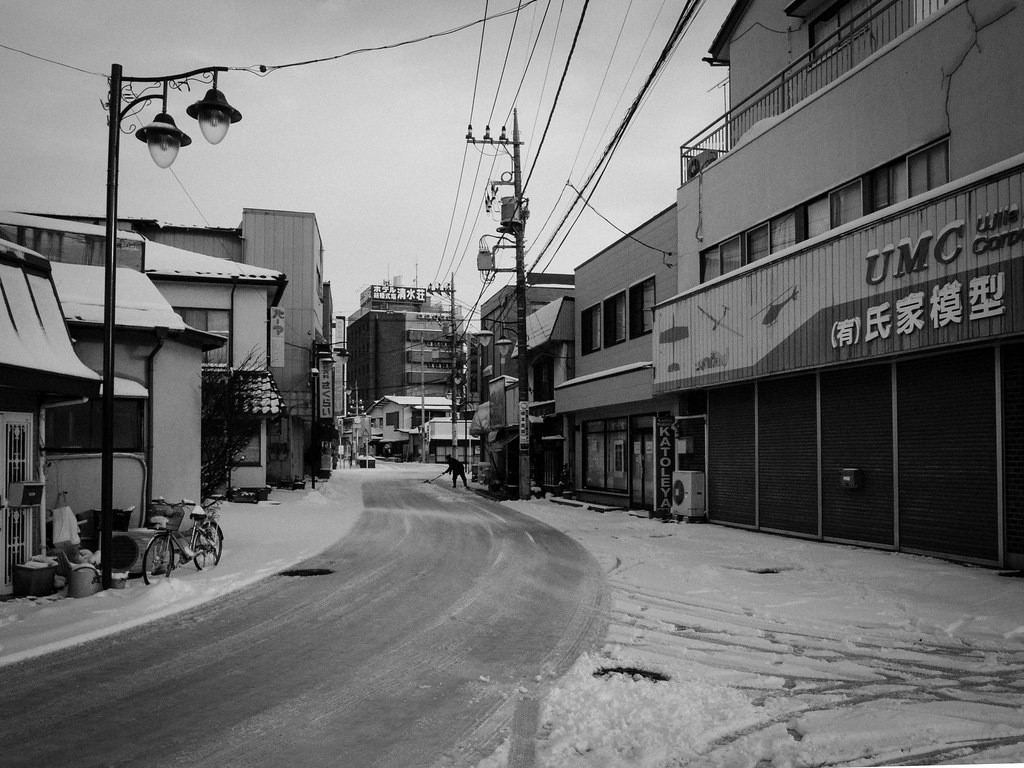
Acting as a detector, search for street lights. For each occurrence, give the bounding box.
[99,63,243,592]
[475,319,532,500]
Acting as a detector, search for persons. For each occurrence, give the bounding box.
[442,454,469,489]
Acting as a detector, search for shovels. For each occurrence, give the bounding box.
[423,472,446,484]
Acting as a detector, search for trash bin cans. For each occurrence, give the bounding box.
[14,559,59,595]
[92,508,133,554]
[368,460,375,468]
[360,460,367,468]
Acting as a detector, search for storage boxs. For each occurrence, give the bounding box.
[12,564,57,597]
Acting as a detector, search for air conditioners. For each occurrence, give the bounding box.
[99,528,156,574]
[428,364,448,368]
[686,148,717,179]
[417,314,446,321]
[427,342,448,347]
[672,470,705,517]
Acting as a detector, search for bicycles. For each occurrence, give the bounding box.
[139,496,225,587]
